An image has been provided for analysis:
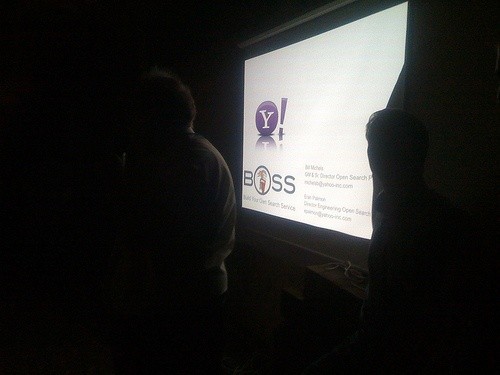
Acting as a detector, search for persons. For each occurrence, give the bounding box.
[129,67,238,374]
[362,110,466,375]
